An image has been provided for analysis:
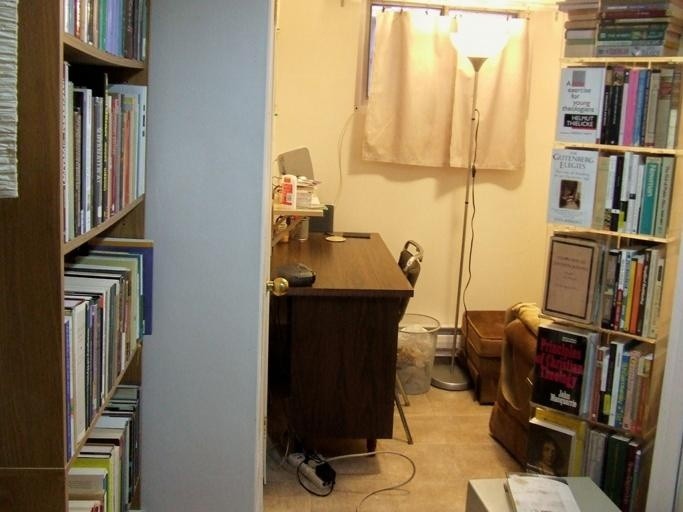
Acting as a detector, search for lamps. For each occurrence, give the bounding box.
[429,29,507,391]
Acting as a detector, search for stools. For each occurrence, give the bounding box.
[460,310,507,405]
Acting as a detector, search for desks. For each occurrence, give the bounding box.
[266,232,413,457]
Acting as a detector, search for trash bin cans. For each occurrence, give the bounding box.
[395,313,441,395]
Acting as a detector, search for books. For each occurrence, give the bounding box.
[523,1,683,512]
[63,62,147,242]
[61,236,156,463]
[505,471,581,512]
[63,0,147,62]
[65,383,142,512]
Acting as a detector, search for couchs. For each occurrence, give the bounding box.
[489,303,555,467]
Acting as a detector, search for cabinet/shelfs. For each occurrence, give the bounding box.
[524,0,683,512]
[0,0,150,512]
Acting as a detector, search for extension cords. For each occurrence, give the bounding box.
[288,450,333,490]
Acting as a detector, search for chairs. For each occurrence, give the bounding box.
[394,239,423,446]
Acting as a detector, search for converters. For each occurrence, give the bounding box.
[316,463,336,483]
